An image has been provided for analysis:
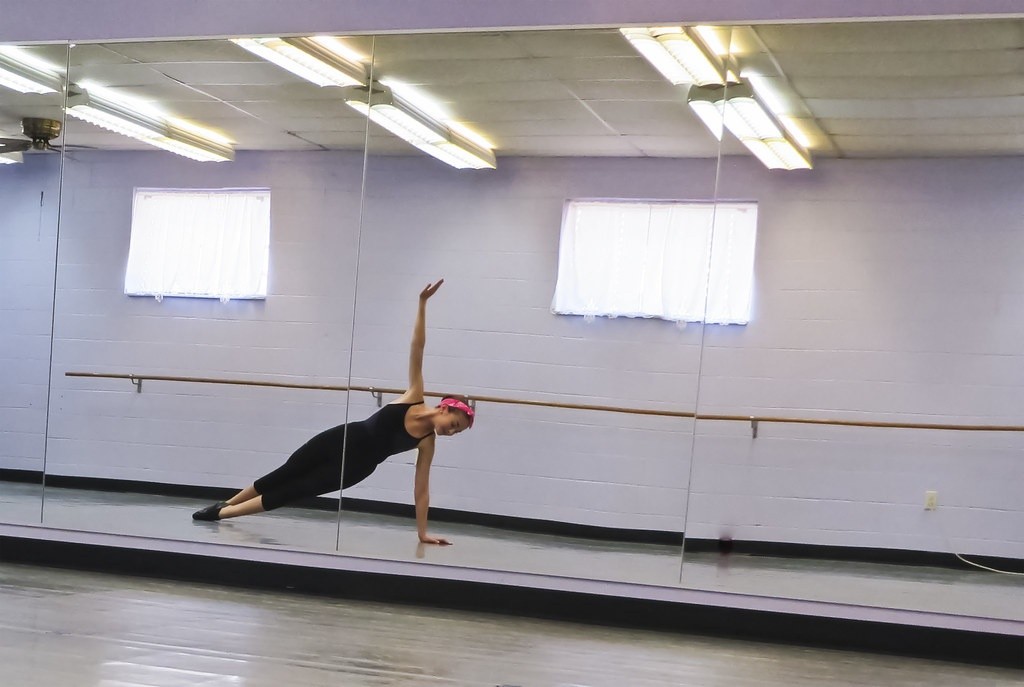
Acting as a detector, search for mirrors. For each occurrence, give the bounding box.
[0,15,1024,622]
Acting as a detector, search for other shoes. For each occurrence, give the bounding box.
[192,501,230,521]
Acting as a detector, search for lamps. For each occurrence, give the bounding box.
[59,88,236,162]
[683,78,813,174]
[0,45,63,95]
[227,36,370,90]
[618,24,742,88]
[343,88,498,171]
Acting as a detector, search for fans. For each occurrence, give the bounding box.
[0,117,99,162]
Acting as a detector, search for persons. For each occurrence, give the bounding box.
[192,279,476,545]
